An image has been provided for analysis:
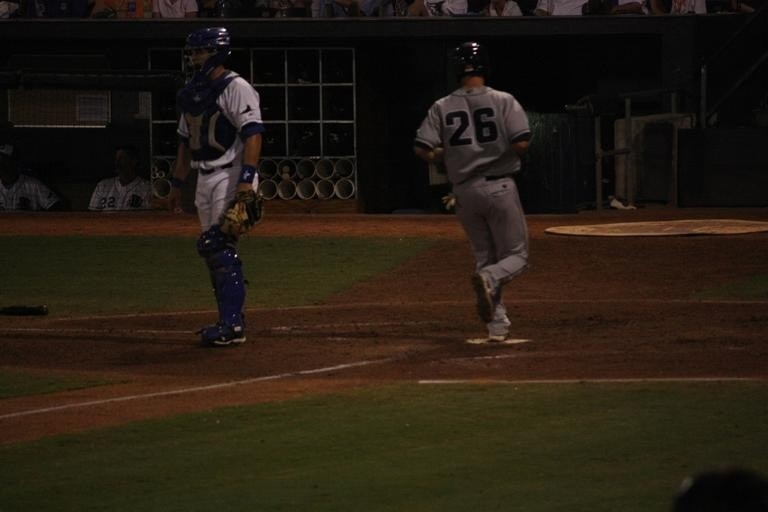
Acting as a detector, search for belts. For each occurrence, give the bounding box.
[199,163,233,174]
[486,176,506,181]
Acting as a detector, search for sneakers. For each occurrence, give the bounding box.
[470,270,501,323]
[209,322,247,346]
[487,323,509,342]
[201,320,224,343]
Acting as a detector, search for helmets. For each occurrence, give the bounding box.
[455,33,493,68]
[180,27,231,88]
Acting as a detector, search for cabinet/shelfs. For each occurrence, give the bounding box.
[146,44,359,199]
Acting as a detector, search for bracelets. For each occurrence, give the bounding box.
[170,176,184,190]
[239,165,255,184]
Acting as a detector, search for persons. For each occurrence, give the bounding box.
[411,36,534,343]
[0,143,66,211]
[87,143,153,211]
[164,27,266,347]
[1,0,767,21]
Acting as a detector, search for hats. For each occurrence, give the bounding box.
[0,143,18,161]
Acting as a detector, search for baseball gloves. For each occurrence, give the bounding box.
[219,189,264,235]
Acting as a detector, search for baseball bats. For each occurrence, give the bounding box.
[1,303,48,316]
